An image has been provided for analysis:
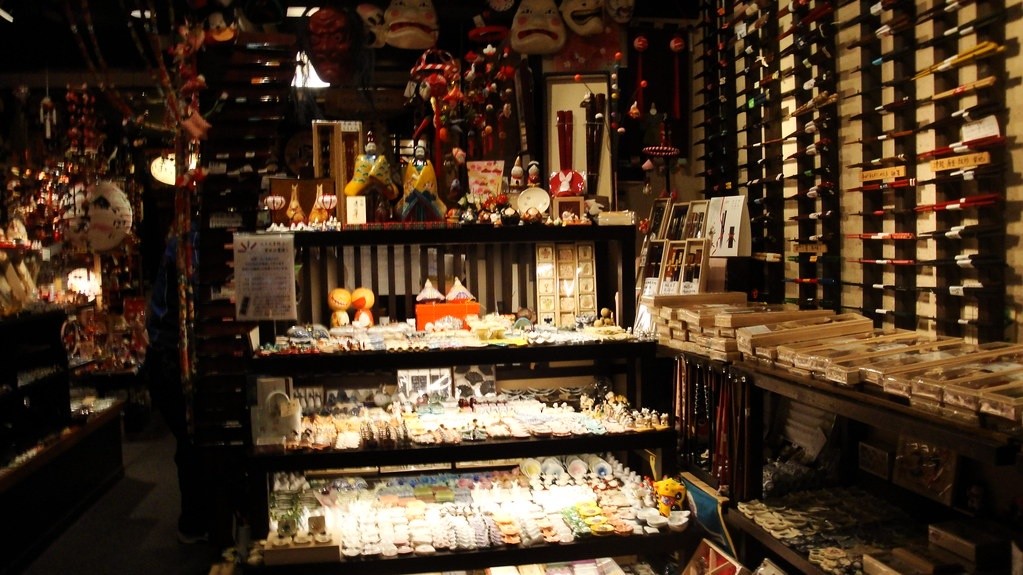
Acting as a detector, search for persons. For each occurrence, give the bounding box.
[344,143,398,221]
[396,146,447,220]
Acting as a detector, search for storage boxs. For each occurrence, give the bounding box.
[535,240,599,333]
[640,199,1023,422]
[682,538,753,575]
[416,300,480,334]
[541,72,617,212]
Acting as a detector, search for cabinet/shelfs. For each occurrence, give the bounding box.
[245,222,682,575]
[654,343,1023,575]
[0,302,126,575]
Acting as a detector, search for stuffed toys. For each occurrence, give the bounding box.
[419,52,515,163]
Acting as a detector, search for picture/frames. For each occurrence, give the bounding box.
[552,197,585,221]
